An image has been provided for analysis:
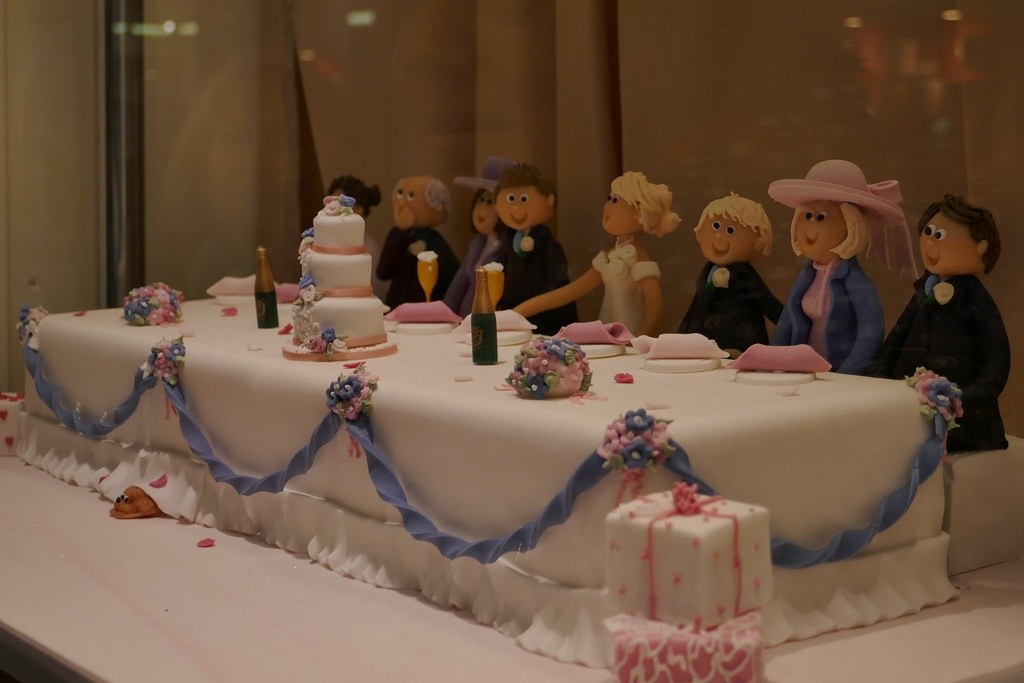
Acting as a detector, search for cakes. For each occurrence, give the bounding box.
[291,210,387,348]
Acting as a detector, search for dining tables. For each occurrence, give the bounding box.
[0,455,1024,682]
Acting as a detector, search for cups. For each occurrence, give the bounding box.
[418,261,437,302]
[486,272,504,310]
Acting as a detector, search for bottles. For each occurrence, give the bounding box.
[471,269,498,364]
[255,245,278,329]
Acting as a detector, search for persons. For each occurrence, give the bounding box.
[444,168,503,320]
[376,175,459,309]
[678,192,783,351]
[329,174,382,219]
[505,171,683,340]
[762,159,920,378]
[874,195,1010,452]
[489,160,580,336]
